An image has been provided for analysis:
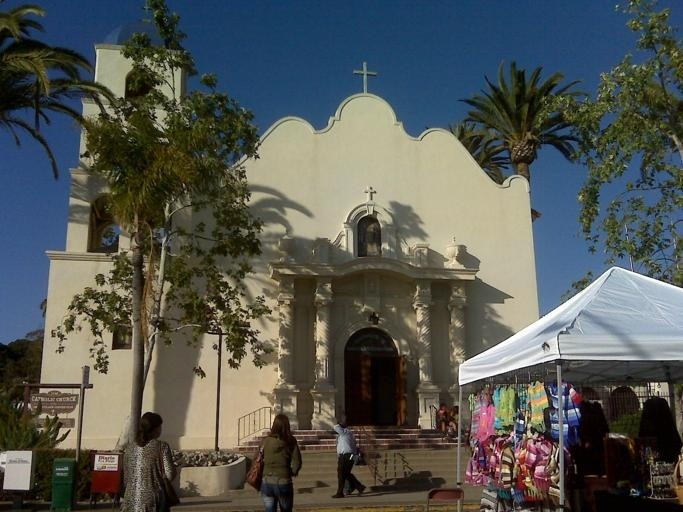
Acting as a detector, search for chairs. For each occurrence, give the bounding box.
[426,488,464,511]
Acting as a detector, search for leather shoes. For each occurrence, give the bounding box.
[332,493,345,498]
[357,485,365,496]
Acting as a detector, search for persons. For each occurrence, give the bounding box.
[252,414,303,512]
[436,403,459,443]
[122,412,176,512]
[332,423,366,499]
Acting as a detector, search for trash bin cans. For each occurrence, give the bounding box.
[52,458,74,512]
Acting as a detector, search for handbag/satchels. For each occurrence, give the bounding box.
[162,473,181,507]
[246,452,265,490]
